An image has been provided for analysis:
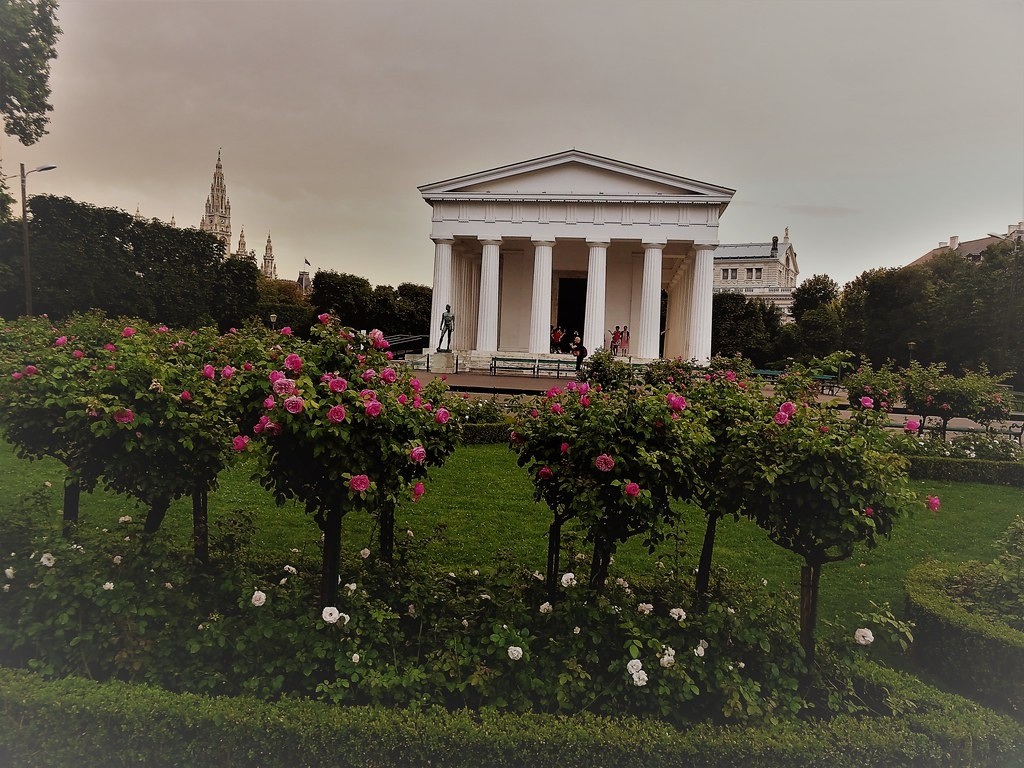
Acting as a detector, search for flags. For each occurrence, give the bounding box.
[305,259,310,265]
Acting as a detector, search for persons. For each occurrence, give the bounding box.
[551,325,581,354]
[608,326,630,357]
[573,337,584,371]
[438,305,455,350]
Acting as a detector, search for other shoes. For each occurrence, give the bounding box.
[558,350,562,353]
[554,351,557,353]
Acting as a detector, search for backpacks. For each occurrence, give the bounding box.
[582,346,588,358]
